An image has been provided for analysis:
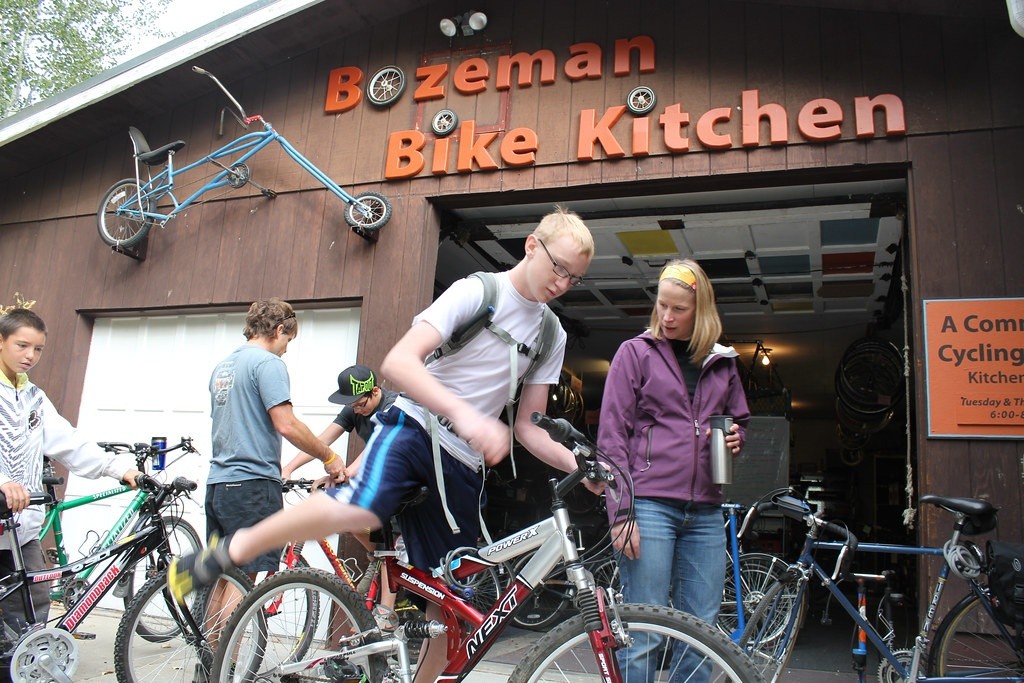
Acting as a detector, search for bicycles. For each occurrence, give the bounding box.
[95,62,394,249]
[207,405,770,683]
[719,491,1024,683]
[1,407,808,683]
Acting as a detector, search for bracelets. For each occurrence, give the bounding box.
[323,452,336,465]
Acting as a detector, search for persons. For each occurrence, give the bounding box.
[0,308,143,652]
[596,260,750,683]
[193,298,346,683]
[168,212,611,683]
[281,364,409,633]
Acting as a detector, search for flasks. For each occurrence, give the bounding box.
[710,416,733,485]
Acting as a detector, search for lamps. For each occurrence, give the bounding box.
[440,7,487,37]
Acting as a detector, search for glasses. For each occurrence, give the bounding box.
[348,400,369,407]
[275,311,297,328]
[533,236,585,289]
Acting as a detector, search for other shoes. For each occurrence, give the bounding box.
[349,603,401,639]
[162,534,217,611]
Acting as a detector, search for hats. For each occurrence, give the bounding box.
[327,364,379,406]
